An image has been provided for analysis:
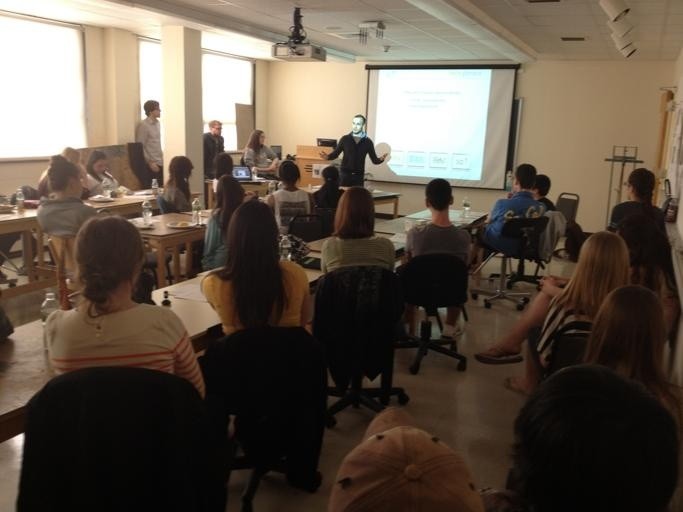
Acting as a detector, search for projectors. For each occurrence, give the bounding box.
[272,44,326,62]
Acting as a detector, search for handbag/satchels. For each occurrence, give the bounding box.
[660,178,679,223]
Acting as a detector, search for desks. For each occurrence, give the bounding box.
[298,185,403,219]
[0,178,217,310]
[235,180,279,197]
[0,262,326,441]
[306,209,490,338]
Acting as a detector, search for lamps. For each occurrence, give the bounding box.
[597,0,636,57]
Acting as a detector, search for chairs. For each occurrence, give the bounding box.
[504,320,683,512]
[313,373,410,430]
[227,422,323,511]
[287,214,328,242]
[395,252,480,376]
[469,192,581,312]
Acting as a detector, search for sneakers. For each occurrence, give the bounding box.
[396,334,419,346]
[441,322,465,338]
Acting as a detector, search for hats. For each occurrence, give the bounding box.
[325,405,488,512]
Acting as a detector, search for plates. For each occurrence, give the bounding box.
[88,197,117,202]
[166,222,198,228]
[135,222,157,229]
[0,204,17,211]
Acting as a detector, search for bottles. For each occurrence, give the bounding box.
[14,185,24,211]
[150,178,158,196]
[461,195,472,220]
[251,165,259,179]
[505,169,512,191]
[162,292,174,309]
[142,197,154,223]
[41,292,60,349]
[268,181,284,197]
[279,234,294,262]
[190,198,203,226]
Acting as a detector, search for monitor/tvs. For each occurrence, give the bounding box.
[317,138,337,146]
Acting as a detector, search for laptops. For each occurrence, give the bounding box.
[232,165,252,181]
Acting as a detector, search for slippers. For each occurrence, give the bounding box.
[473,345,524,364]
[503,374,540,398]
